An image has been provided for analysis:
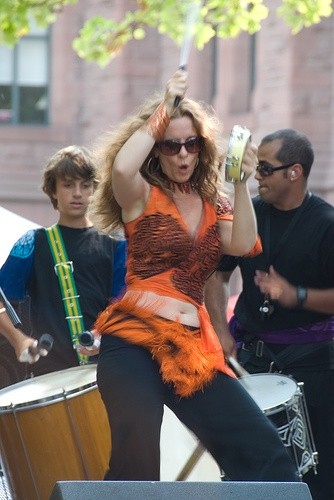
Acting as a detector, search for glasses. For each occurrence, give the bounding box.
[153,135,204,156]
[256,164,294,177]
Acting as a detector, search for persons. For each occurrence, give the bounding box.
[203,128,334,500]
[90,71,300,482]
[0,146,127,380]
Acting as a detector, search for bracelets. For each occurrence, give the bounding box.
[140,103,171,144]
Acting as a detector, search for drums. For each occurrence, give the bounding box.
[220,372,319,482]
[225,125,252,183]
[0,363,112,500]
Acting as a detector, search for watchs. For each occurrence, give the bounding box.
[296,284,308,310]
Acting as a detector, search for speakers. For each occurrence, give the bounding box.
[49,480,313,500]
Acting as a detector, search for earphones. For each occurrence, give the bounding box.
[291,170,295,177]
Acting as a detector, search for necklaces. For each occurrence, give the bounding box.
[163,174,193,195]
